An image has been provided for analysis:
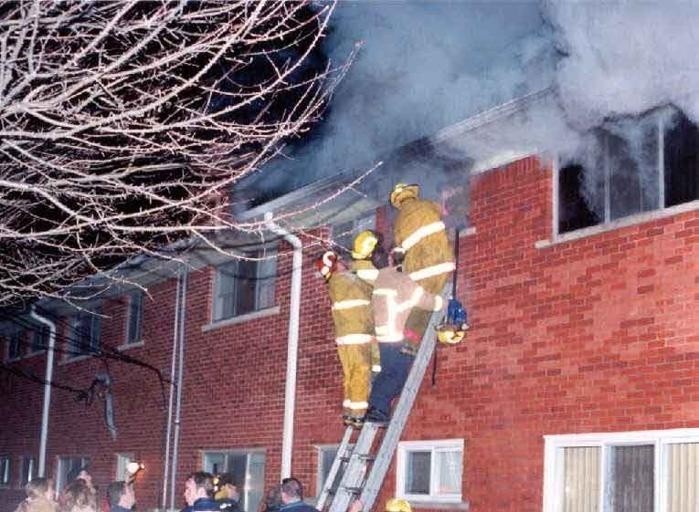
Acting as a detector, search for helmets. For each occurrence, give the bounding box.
[385,497,411,512]
[390,183,419,210]
[351,231,377,260]
[317,250,343,285]
[434,322,469,343]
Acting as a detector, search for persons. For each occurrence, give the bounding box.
[182,471,240,511]
[349,228,380,283]
[385,497,411,510]
[16,469,135,511]
[364,247,446,427]
[327,489,362,510]
[390,182,456,355]
[260,477,317,511]
[316,249,373,428]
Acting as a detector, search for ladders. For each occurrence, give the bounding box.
[315,282,453,512]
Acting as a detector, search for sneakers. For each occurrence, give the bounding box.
[399,336,420,356]
[341,407,390,430]
